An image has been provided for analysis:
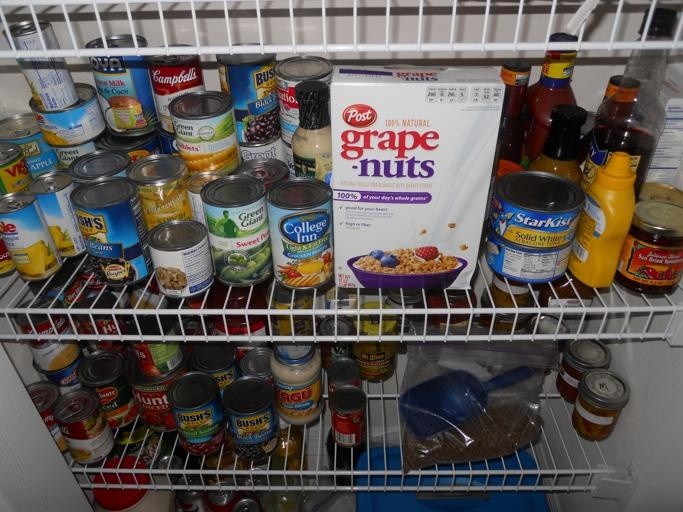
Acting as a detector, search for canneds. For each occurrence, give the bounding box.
[488,171,583,280]
[10,260,631,512]
[0,21,334,297]
[613,182,682,296]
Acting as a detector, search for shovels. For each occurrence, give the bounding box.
[399,365,538,438]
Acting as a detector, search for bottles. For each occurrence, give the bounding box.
[290,81,333,184]
[499,5,677,289]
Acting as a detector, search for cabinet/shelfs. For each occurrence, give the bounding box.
[0,1,683,512]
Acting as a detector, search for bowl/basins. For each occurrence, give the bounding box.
[346,253,468,292]
